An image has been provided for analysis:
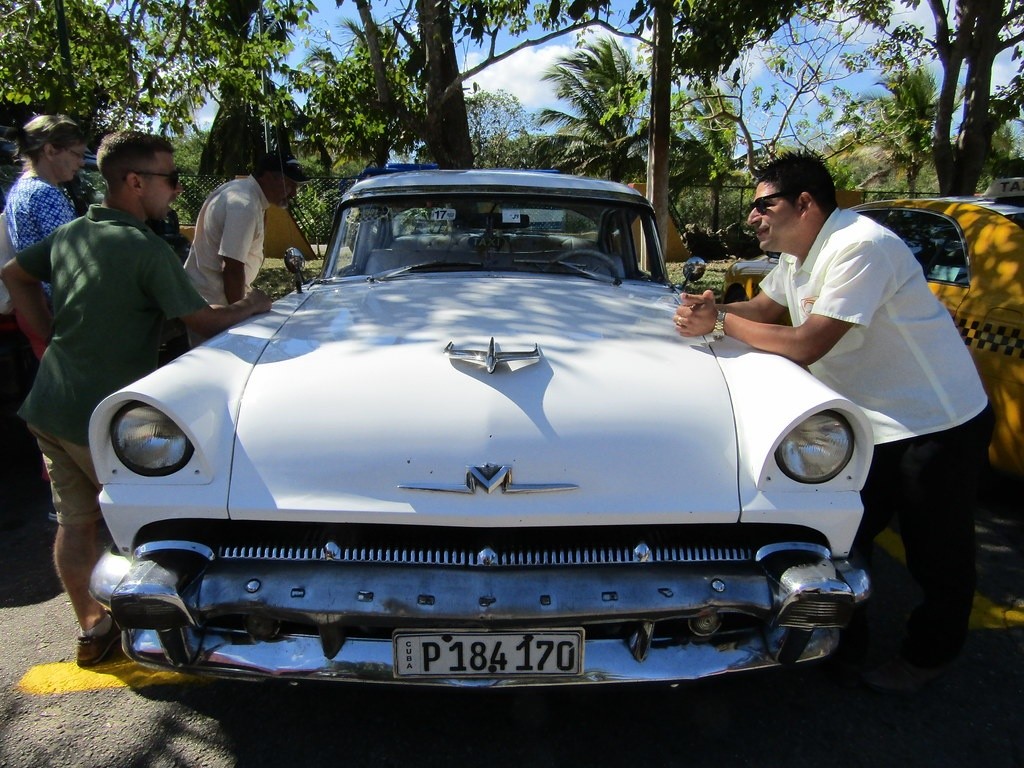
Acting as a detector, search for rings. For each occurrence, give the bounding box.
[675,315,685,326]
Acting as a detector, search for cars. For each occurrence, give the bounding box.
[86,166,875,692]
[720,177,1024,478]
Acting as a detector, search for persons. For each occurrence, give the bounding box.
[673,143,999,695]
[0,129,274,669]
[178,150,314,351]
[3,110,104,523]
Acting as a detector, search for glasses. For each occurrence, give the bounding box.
[121,170,179,190]
[749,191,790,214]
[65,144,86,160]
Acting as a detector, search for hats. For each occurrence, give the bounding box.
[251,145,311,185]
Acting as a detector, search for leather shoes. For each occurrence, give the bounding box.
[76,609,125,667]
[861,654,964,697]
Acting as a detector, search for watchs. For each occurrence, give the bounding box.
[709,307,727,342]
